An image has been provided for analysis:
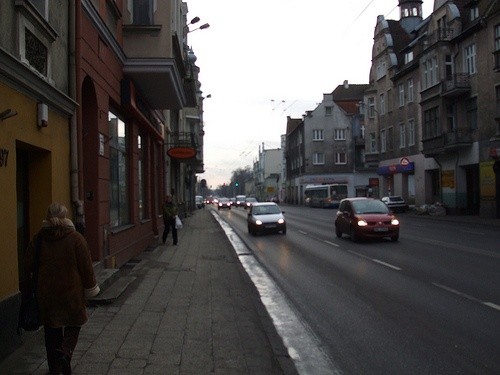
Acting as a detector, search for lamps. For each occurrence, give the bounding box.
[38,101,48,130]
[183,16,200,28]
[188,23,210,32]
[199,94,211,101]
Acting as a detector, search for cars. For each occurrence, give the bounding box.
[247,202,287,236]
[195,195,257,211]
[381,196,405,212]
[334,197,400,242]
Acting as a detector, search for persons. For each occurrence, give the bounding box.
[24,203,99,375]
[161,194,178,246]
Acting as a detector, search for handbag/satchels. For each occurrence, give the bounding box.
[18,281,40,332]
[175,214,183,229]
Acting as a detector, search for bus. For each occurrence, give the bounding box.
[304,184,349,209]
